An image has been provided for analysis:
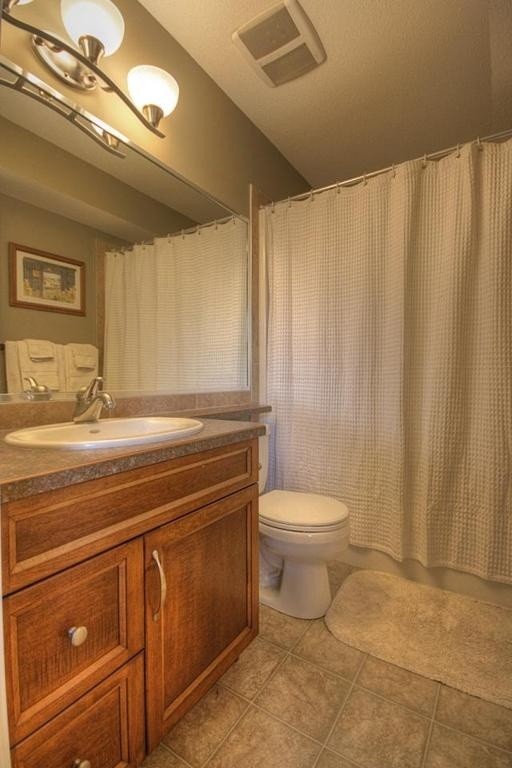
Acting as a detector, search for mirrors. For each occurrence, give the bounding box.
[1,56,258,406]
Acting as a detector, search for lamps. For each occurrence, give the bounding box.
[57,0,182,143]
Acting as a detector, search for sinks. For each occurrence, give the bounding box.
[2,416,205,452]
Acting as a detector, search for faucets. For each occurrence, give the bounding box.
[72,376,112,422]
[21,374,52,400]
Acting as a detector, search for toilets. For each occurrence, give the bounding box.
[258,421,352,620]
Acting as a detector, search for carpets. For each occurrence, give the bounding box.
[323,570,511,711]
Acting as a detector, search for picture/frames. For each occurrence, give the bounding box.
[10,238,89,314]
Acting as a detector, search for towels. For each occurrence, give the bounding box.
[4,338,98,394]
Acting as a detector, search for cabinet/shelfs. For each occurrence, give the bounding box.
[2,439,258,768]
